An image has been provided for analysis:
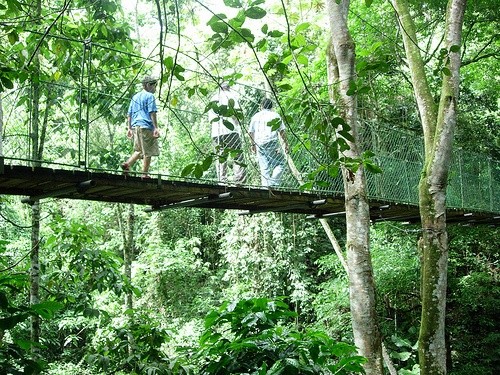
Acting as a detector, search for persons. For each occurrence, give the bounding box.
[119,74,161,181]
[249,97,292,188]
[209,80,251,187]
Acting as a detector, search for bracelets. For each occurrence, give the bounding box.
[251,143,255,146]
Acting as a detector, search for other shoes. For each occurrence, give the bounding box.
[121,162,131,176]
